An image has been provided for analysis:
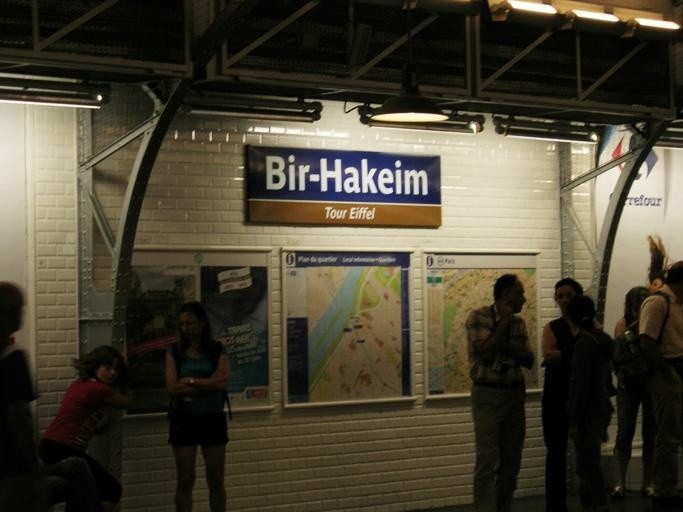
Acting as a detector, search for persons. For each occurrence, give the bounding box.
[466,273,533,510]
[0,280,42,510]
[564,295,617,511]
[541,277,610,509]
[645,232,668,294]
[606,287,657,498]
[638,259,682,512]
[164,298,230,510]
[42,348,134,510]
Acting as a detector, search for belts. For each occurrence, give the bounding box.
[472,380,524,389]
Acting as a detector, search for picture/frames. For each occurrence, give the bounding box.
[122,242,276,419]
[419,247,544,401]
[278,243,420,409]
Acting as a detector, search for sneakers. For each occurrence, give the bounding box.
[608,485,657,498]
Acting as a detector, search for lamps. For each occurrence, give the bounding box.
[176,92,324,124]
[356,101,487,136]
[490,0,681,41]
[1,80,114,112]
[489,112,682,151]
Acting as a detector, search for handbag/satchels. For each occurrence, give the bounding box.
[168,390,228,428]
[608,292,671,390]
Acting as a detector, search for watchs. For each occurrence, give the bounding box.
[188,375,195,389]
